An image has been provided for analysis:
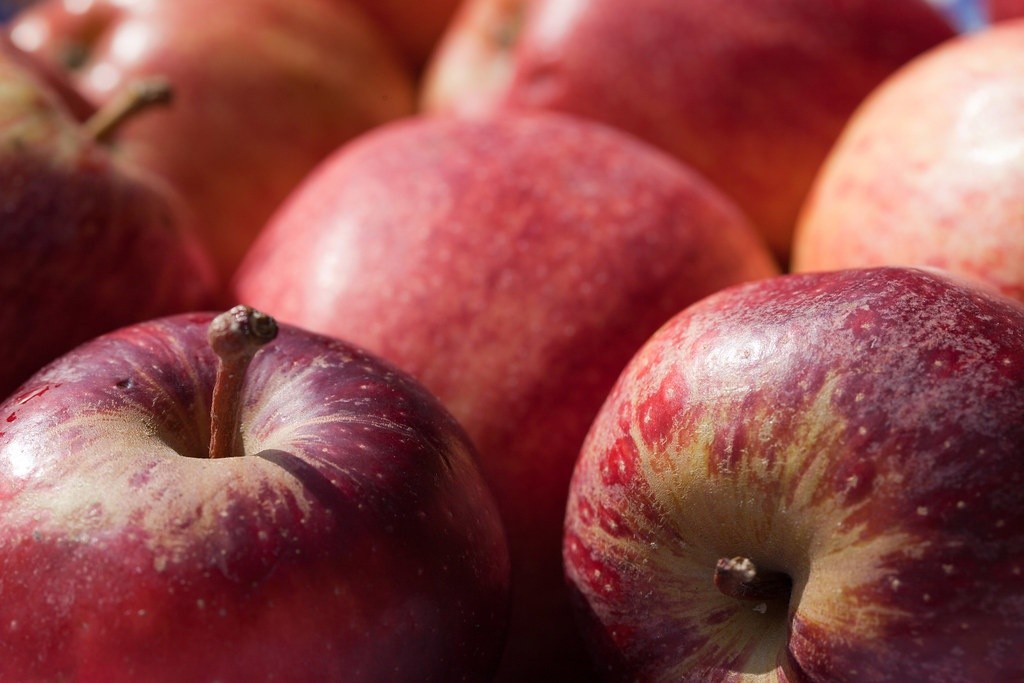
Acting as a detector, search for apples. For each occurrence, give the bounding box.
[0,0,1024,683]
